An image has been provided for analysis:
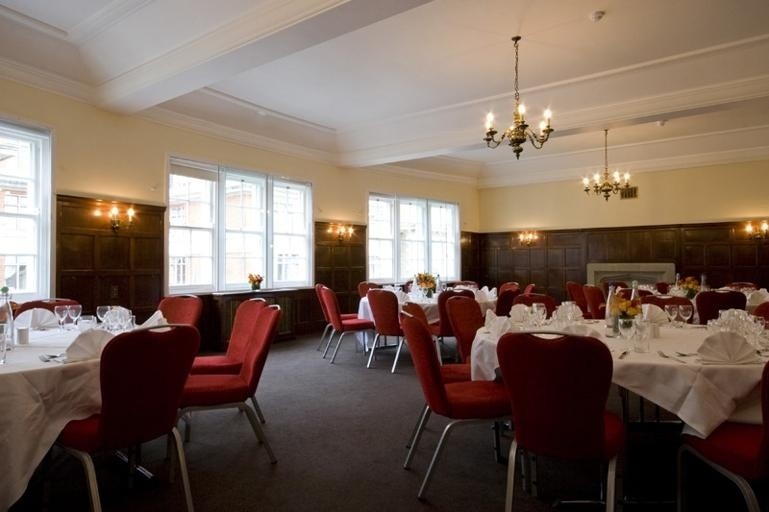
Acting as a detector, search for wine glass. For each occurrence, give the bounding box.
[52,303,136,341]
[522,298,765,356]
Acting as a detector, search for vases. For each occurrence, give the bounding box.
[251,283,260,289]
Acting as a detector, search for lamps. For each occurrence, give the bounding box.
[481,32,554,160]
[582,128,631,201]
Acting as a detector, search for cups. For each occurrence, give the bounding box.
[1,322,10,364]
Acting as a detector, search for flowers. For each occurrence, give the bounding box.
[248,274,263,285]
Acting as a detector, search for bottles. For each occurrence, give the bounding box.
[603,278,641,337]
[411,271,443,298]
[0,294,16,353]
[674,272,707,292]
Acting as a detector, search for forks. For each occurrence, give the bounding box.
[655,350,685,365]
